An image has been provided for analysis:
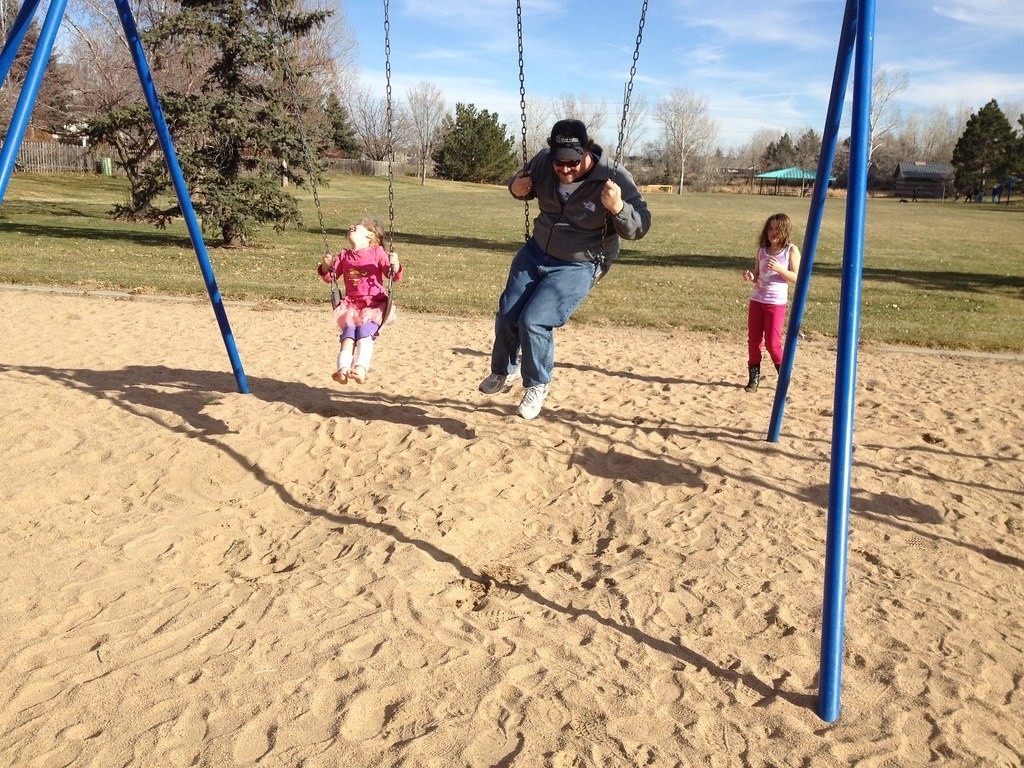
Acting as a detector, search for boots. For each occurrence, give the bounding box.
[775,365,780,374]
[745,365,760,393]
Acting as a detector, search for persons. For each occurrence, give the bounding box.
[898,174,1008,206]
[315,216,404,384]
[741,211,803,394]
[478,119,653,420]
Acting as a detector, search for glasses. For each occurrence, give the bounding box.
[553,159,581,167]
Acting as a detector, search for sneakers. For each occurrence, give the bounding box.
[516,383,551,420]
[478,367,521,393]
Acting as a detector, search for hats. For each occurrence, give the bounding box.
[550,119,592,160]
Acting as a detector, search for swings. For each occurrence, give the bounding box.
[514,0,652,290]
[268,0,396,332]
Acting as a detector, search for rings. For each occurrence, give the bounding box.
[528,185,531,190]
